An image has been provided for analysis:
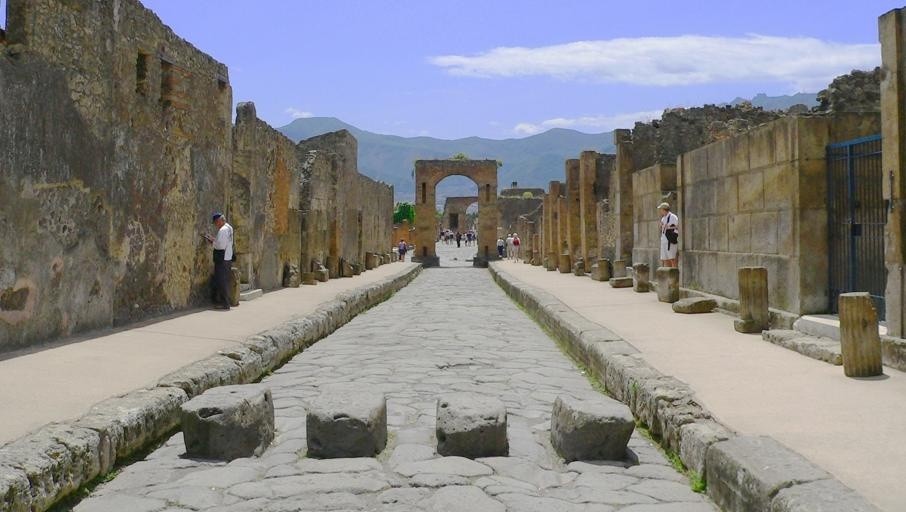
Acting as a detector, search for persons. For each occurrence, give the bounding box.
[404,242,409,253]
[206,213,233,310]
[497,236,504,258]
[657,202,679,267]
[511,233,520,263]
[506,234,513,260]
[399,239,405,262]
[440,229,476,247]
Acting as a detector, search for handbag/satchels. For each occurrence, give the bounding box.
[665,214,678,251]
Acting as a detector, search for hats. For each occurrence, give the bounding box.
[212,214,221,224]
[499,233,517,239]
[657,202,670,210]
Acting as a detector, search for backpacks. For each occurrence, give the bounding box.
[513,238,520,246]
